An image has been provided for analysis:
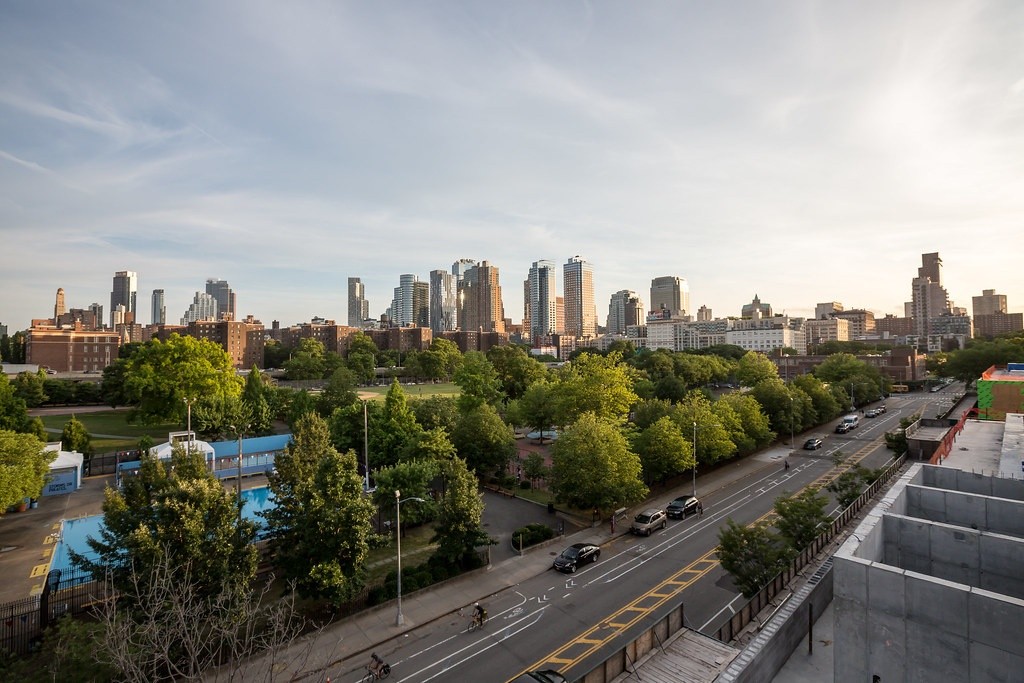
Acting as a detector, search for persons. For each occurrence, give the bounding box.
[516,464,522,481]
[472,603,486,631]
[697,498,704,517]
[784,459,789,473]
[368,652,384,683]
[858,408,866,417]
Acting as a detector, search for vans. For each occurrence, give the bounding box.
[836,415,859,434]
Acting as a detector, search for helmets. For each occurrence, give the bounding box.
[473,602,479,606]
[371,652,378,659]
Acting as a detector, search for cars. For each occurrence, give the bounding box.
[509,669,568,683]
[804,438,823,450]
[553,543,601,573]
[865,405,887,418]
[930,377,955,392]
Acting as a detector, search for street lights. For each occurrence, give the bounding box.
[693,422,696,495]
[183,397,196,456]
[396,490,424,627]
[230,424,251,539]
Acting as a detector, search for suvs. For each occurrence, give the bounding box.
[665,495,700,520]
[629,509,667,536]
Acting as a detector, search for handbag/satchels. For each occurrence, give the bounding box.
[482,608,488,619]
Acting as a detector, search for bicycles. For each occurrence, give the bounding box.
[468,613,489,633]
[362,664,391,683]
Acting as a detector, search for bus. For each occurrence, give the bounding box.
[890,385,909,394]
